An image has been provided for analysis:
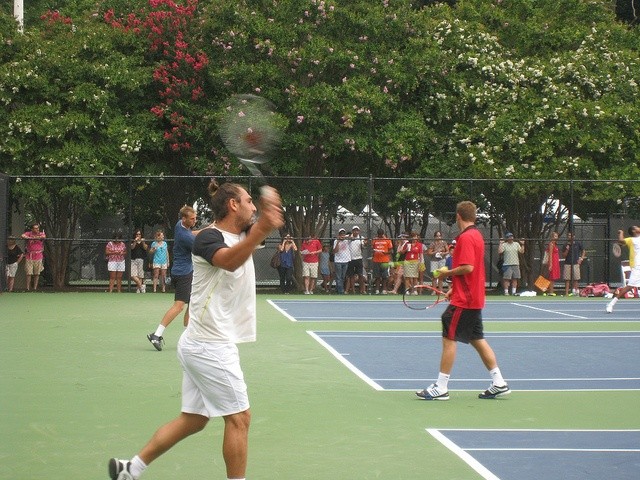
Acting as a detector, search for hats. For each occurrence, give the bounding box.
[378,229,384,235]
[352,226,360,230]
[506,233,513,237]
[339,229,346,232]
[398,234,409,238]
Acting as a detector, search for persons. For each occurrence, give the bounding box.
[6,236,24,291]
[109,179,286,480]
[562,232,585,298]
[448,239,456,247]
[606,226,640,313]
[548,231,560,295]
[416,201,511,400]
[106,234,126,292]
[416,234,429,294]
[21,224,46,291]
[301,235,322,295]
[445,247,455,300]
[277,233,298,295]
[129,230,147,294]
[320,246,332,294]
[498,232,524,296]
[428,231,449,295]
[389,231,412,295]
[346,226,368,294]
[401,231,420,296]
[146,206,200,352]
[371,229,393,294]
[333,228,353,294]
[150,228,170,293]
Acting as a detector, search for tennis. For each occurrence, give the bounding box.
[433,270,441,278]
[440,267,448,271]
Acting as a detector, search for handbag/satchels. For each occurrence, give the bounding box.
[165,267,171,286]
[270,246,281,268]
[536,275,550,291]
[542,250,549,264]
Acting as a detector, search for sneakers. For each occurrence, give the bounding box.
[416,383,450,401]
[543,293,546,296]
[375,290,379,294]
[109,458,134,480]
[381,290,387,294]
[414,291,418,295]
[406,291,409,295]
[141,285,146,292]
[606,303,613,312]
[504,293,508,295]
[548,293,556,296]
[136,289,140,292]
[511,293,517,296]
[479,384,510,399]
[147,333,165,351]
